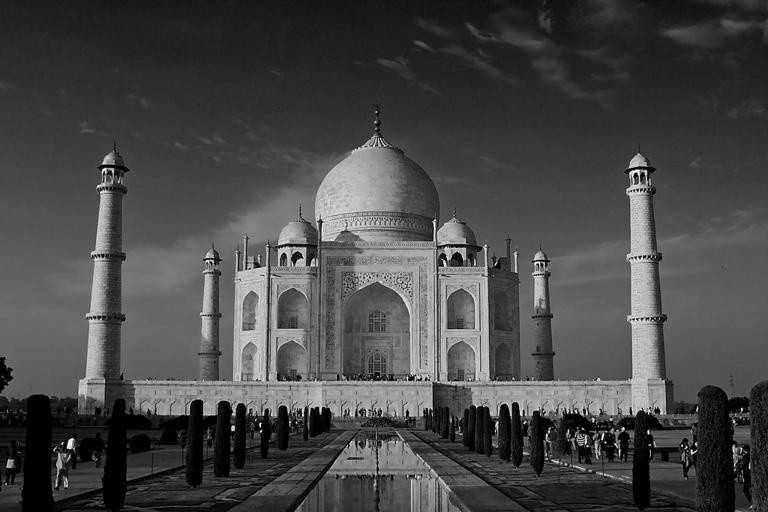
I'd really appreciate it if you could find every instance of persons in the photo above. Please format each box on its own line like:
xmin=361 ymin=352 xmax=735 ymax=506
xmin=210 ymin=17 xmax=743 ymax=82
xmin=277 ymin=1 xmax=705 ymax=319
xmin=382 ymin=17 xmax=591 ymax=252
xmin=211 ymin=425 xmax=217 ymax=442
xmin=447 ymin=406 xmax=632 ymax=463
xmin=392 ymin=408 xmax=397 ymax=417
xmin=678 ymin=418 xmax=754 ymax=512
xmin=342 ymin=406 xmax=384 ymax=418
xmin=230 ymin=423 xmax=235 ymax=440
xmin=62 ymin=404 xmax=158 ymax=415
xmin=640 ymin=406 xmax=661 ymax=415
xmin=65 ymin=433 xmax=79 ymax=469
xmin=3 ymin=438 xmax=21 ymax=484
xmin=466 ymin=374 xmax=543 ymax=382
xmin=646 ymin=429 xmax=657 ymax=462
xmin=591 ymin=375 xmax=601 ymax=382
xmin=53 ymin=440 xmax=73 ymax=491
xmin=247 ymin=416 xmax=304 ymax=440
xmin=405 ymin=408 xmax=410 ymax=417
xmin=282 ymin=371 xmax=432 ymax=381
xmin=91 ymin=430 xmax=103 ymax=467
xmin=205 ymin=426 xmax=213 ymax=448
